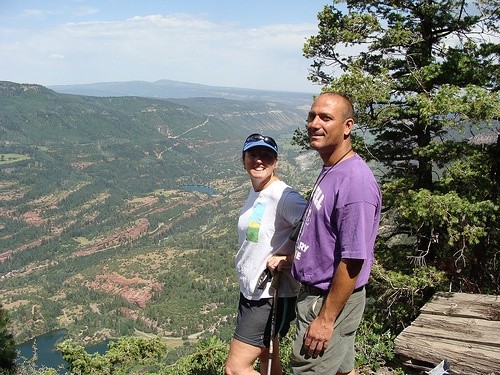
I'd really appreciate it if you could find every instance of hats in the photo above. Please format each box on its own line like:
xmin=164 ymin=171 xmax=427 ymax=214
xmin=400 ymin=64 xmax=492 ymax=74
xmin=242 ymin=137 xmax=279 ymax=154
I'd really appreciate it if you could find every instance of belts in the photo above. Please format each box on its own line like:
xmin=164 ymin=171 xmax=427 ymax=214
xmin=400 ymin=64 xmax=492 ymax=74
xmin=304 ymin=285 xmax=364 ymax=296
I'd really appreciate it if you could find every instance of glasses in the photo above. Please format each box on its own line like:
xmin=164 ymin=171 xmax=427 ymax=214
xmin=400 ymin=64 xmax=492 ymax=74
xmin=246 ymin=133 xmax=278 ymax=149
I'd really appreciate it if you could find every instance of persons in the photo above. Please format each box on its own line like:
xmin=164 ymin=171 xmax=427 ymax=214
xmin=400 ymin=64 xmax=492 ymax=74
xmin=226 ymin=136 xmax=308 ymax=375
xmin=286 ymin=92 xmax=382 ymax=375
